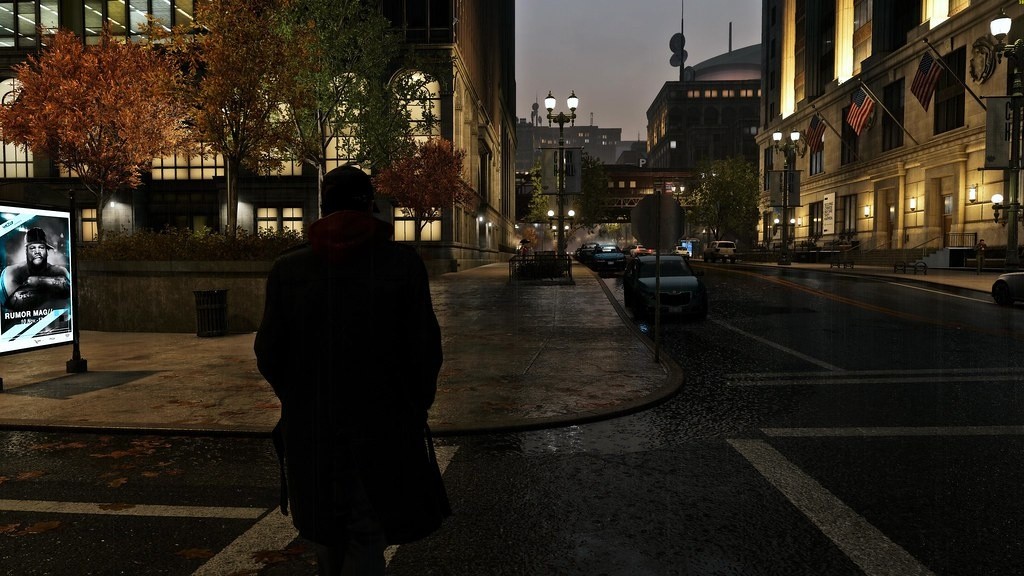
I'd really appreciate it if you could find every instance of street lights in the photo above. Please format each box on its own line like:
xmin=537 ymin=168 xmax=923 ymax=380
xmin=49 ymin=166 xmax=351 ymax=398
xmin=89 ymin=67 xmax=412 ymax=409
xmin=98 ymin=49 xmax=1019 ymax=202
xmin=773 ymin=127 xmax=800 ymax=265
xmin=543 ymin=87 xmax=578 ymax=283
xmin=989 ymin=7 xmax=1024 ymax=274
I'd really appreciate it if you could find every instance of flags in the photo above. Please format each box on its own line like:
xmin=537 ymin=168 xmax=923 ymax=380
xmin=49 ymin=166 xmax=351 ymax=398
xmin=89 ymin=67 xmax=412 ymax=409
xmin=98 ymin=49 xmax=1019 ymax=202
xmin=805 ymin=114 xmax=827 ymax=156
xmin=910 ymin=50 xmax=945 ymax=112
xmin=845 ymin=87 xmax=875 ymax=137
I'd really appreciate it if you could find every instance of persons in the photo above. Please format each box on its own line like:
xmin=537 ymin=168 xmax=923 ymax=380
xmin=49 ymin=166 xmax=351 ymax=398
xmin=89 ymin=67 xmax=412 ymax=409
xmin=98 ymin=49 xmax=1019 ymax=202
xmin=253 ymin=166 xmax=443 ymax=576
xmin=974 ymin=239 xmax=988 ymax=275
xmin=520 ymin=243 xmax=529 ymax=254
xmin=0 ymin=227 xmax=70 ymax=330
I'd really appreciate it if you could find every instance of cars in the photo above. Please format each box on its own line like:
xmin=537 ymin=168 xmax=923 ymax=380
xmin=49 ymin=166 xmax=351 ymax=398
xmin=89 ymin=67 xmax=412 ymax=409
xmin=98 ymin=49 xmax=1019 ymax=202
xmin=675 ymin=247 xmax=689 ymax=260
xmin=704 ymin=241 xmax=737 ymax=264
xmin=630 ymin=244 xmax=652 ymax=255
xmin=622 ymin=255 xmax=704 ymax=323
xmin=576 ymin=241 xmax=626 ymax=272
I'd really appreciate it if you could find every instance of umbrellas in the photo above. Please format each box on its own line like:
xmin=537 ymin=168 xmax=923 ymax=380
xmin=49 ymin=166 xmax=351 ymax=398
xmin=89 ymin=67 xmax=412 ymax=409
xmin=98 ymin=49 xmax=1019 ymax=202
xmin=520 ymin=239 xmax=530 ymax=243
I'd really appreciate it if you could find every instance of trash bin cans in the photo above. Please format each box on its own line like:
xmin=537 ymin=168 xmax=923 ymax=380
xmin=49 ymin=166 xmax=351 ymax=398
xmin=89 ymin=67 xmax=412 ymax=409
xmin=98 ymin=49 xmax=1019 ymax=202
xmin=190 ymin=286 xmax=231 ymax=338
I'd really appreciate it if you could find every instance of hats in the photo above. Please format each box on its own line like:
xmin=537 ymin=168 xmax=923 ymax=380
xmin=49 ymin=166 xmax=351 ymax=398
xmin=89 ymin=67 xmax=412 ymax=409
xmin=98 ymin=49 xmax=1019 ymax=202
xmin=24 ymin=227 xmax=55 ymax=250
xmin=520 ymin=239 xmax=530 ymax=244
